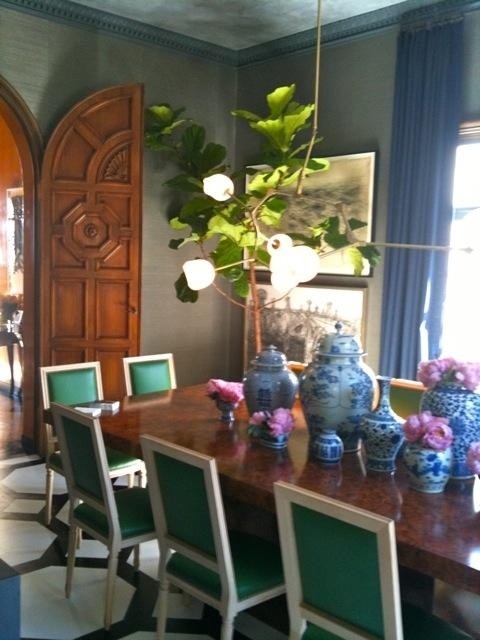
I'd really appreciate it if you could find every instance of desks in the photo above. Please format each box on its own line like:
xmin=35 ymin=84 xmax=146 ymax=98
xmin=1 ymin=332 xmax=20 ymax=399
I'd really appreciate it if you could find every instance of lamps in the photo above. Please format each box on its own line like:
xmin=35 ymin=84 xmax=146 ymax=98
xmin=183 ymin=170 xmax=322 ymax=312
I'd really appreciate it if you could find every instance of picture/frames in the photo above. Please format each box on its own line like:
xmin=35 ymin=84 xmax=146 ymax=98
xmin=241 ymin=275 xmax=370 ymax=372
xmin=238 ymin=151 xmax=379 ymax=278
